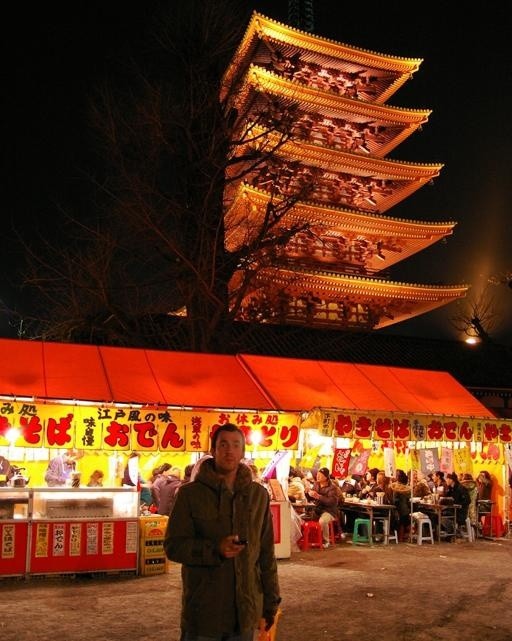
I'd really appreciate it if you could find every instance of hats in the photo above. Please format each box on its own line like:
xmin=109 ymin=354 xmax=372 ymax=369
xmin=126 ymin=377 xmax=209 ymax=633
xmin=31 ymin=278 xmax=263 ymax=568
xmin=318 ymin=468 xmax=328 ymax=478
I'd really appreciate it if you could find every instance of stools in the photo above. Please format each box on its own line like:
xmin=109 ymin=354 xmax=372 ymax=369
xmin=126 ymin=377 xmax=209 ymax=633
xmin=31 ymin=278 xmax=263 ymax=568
xmin=296 ymin=512 xmax=506 ymax=555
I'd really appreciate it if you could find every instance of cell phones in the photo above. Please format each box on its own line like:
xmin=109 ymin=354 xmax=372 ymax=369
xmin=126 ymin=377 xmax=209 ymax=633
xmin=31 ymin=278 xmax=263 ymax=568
xmin=234 ymin=539 xmax=249 ymax=545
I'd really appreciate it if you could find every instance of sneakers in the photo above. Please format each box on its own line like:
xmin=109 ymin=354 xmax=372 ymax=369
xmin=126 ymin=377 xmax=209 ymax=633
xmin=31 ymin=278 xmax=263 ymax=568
xmin=322 ymin=543 xmax=330 ymax=549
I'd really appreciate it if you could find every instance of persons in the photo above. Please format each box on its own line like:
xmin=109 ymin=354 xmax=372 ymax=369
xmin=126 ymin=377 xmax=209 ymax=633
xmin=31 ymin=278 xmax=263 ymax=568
xmin=45 ymin=448 xmax=85 ymax=489
xmin=0 ymin=454 xmax=20 ymax=523
xmin=162 ymin=424 xmax=284 ymax=641
xmin=250 ymin=465 xmax=493 ymax=549
xmin=124 ymin=452 xmax=215 ymax=517
xmin=85 ymin=470 xmax=105 ymax=488
